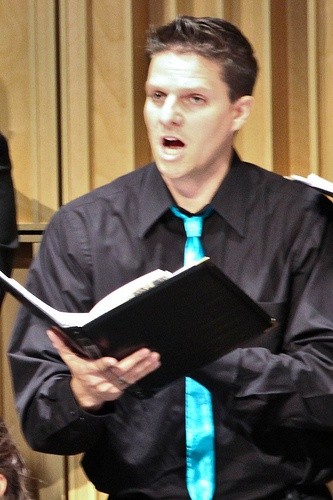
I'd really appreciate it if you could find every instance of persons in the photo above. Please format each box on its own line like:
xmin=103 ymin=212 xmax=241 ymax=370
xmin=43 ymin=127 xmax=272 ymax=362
xmin=0 ymin=129 xmax=19 ymax=312
xmin=7 ymin=16 xmax=333 ymax=500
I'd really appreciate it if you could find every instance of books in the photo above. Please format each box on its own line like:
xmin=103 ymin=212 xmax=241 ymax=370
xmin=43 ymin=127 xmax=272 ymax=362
xmin=0 ymin=254 xmax=276 ymax=401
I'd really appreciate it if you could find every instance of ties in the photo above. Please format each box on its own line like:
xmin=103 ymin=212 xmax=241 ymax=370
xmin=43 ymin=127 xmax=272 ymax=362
xmin=169 ymin=206 xmax=215 ymax=500
